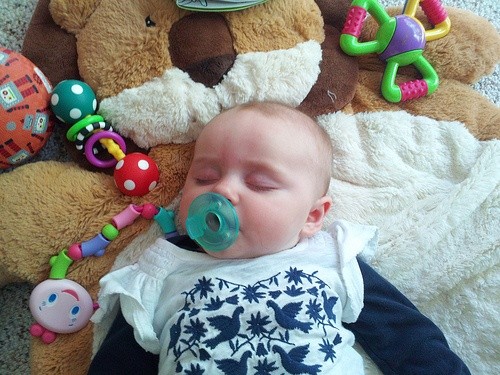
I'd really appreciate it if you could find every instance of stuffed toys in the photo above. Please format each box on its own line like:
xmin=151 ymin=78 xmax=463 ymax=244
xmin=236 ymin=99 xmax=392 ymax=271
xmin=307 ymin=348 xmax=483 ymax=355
xmin=1 ymin=0 xmax=500 ymax=375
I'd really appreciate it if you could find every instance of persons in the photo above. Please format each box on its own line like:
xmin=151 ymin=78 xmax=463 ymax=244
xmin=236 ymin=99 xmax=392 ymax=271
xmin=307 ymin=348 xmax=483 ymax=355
xmin=86 ymin=101 xmax=471 ymax=375
